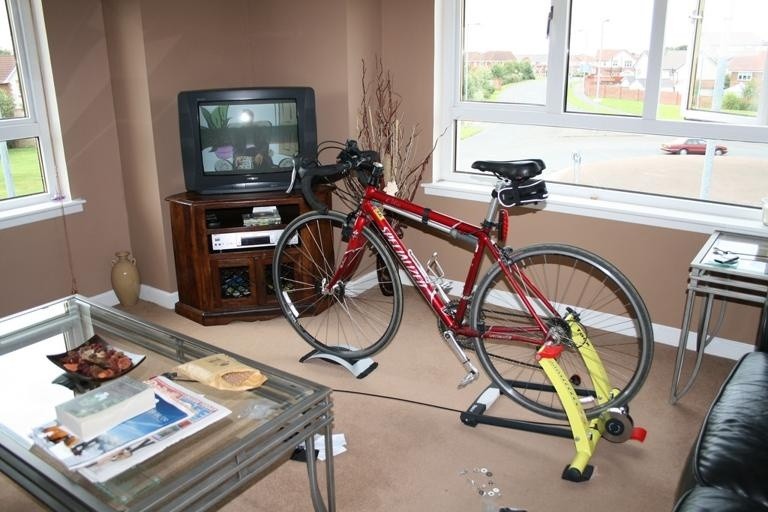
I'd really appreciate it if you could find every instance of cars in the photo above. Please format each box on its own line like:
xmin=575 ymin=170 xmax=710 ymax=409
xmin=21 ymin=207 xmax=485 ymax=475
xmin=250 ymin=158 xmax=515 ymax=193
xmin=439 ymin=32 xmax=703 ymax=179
xmin=659 ymin=138 xmax=728 ymax=156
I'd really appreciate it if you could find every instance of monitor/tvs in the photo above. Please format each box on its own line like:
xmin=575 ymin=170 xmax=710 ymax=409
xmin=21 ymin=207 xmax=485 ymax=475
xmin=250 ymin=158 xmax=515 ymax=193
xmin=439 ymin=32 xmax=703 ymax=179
xmin=178 ymin=85 xmax=319 ymax=194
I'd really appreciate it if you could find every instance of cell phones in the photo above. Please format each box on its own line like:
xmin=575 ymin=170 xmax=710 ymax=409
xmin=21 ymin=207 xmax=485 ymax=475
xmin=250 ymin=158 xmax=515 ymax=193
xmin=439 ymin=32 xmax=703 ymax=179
xmin=715 ymin=253 xmax=739 ymax=263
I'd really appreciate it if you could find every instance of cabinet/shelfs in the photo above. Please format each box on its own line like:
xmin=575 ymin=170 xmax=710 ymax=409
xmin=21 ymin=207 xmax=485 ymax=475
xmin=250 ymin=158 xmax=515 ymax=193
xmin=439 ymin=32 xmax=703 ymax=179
xmin=164 ymin=186 xmax=339 ymax=326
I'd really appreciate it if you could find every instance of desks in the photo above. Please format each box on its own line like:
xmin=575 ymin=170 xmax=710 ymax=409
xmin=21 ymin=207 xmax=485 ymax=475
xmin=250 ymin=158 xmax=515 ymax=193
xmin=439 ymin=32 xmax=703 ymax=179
xmin=668 ymin=229 xmax=768 ymax=406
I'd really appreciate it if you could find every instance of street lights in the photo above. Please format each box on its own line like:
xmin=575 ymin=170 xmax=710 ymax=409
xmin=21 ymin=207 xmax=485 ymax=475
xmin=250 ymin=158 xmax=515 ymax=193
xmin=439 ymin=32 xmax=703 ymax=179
xmin=593 ymin=18 xmax=611 ymax=107
xmin=464 ymin=23 xmax=481 ymax=101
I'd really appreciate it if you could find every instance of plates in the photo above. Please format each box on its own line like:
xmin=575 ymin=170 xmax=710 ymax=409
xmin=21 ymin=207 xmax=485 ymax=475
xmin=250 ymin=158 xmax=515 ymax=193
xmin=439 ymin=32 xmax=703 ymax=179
xmin=48 ymin=336 xmax=144 ymax=379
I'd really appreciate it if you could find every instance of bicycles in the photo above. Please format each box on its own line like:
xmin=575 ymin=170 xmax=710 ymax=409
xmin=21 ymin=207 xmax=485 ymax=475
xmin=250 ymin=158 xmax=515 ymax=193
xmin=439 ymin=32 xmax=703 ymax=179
xmin=271 ymin=139 xmax=654 ymax=421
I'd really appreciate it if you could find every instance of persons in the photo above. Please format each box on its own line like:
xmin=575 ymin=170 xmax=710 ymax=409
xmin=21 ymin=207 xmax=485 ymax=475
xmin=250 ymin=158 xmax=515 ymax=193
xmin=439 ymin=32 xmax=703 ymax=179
xmin=232 ymin=107 xmax=274 ymax=170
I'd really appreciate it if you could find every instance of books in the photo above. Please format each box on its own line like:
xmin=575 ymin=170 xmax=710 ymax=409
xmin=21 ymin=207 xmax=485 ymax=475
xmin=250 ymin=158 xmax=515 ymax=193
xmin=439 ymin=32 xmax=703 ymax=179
xmin=30 ymin=385 xmax=193 ymax=472
xmin=54 ymin=373 xmax=157 ymax=442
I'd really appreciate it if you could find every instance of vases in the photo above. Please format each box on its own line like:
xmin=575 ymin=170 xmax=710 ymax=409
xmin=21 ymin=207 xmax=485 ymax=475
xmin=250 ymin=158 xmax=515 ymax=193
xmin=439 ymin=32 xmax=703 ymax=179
xmin=376 ymin=215 xmax=407 ymax=296
xmin=109 ymin=251 xmax=142 ymax=307
